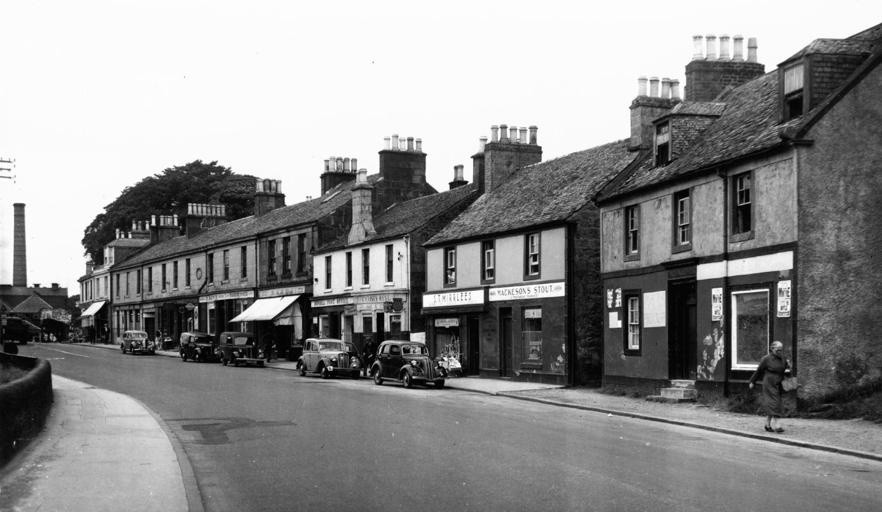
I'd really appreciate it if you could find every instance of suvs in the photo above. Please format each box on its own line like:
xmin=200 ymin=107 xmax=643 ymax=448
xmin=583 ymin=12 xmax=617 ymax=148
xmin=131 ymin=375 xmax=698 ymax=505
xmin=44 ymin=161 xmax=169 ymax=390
xmin=178 ymin=331 xmax=220 ymax=363
xmin=294 ymin=336 xmax=362 ymax=380
xmin=119 ymin=329 xmax=157 ymax=356
xmin=370 ymin=337 xmax=446 ymax=389
xmin=218 ymin=329 xmax=266 ymax=367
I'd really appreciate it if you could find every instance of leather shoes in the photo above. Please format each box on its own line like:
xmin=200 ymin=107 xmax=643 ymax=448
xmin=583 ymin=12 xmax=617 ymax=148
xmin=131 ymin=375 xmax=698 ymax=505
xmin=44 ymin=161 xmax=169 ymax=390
xmin=764 ymin=425 xmax=783 ymax=433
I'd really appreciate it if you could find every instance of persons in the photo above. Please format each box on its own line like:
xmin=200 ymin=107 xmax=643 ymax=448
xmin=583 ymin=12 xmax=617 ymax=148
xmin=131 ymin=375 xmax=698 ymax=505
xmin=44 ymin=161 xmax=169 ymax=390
xmin=748 ymin=340 xmax=793 ymax=434
xmin=155 ymin=327 xmax=163 ymax=350
xmin=90 ymin=324 xmax=96 ymax=344
xmin=102 ymin=323 xmax=110 ymax=344
xmin=362 ymin=337 xmax=376 ymax=377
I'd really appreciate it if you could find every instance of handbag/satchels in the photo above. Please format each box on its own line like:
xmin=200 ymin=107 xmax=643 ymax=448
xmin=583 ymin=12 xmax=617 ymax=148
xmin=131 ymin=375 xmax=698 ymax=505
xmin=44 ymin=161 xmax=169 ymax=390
xmin=782 ymin=376 xmax=798 ymax=391
